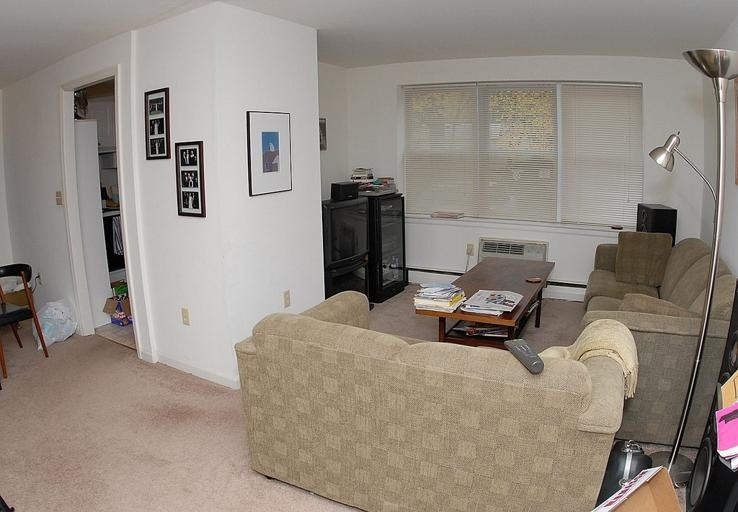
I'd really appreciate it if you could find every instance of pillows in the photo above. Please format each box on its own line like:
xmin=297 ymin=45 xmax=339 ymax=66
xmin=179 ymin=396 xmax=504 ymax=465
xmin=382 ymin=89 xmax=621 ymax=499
xmin=616 ymin=231 xmax=672 ymax=287
xmin=618 ymin=293 xmax=702 ymax=319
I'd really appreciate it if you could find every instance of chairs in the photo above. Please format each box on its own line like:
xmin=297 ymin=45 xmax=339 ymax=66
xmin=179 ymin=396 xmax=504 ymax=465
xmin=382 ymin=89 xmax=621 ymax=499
xmin=0 ymin=263 xmax=49 ymax=378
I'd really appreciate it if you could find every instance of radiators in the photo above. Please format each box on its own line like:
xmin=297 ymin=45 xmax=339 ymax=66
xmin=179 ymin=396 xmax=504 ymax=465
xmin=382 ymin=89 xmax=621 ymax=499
xmin=477 ymin=237 xmax=549 ymax=264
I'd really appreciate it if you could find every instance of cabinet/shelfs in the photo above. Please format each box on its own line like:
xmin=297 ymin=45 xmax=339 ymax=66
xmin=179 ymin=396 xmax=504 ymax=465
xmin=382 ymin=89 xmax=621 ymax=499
xmin=325 ymin=263 xmax=374 ymax=311
xmin=359 ymin=191 xmax=409 ymax=303
xmin=73 ymin=97 xmax=126 ymax=329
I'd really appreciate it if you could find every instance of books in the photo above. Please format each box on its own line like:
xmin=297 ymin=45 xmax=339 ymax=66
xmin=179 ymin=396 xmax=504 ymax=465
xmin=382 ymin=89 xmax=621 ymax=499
xmin=348 ymin=167 xmax=399 ymax=197
xmin=431 ymin=211 xmax=464 ymax=219
xmin=412 ymin=281 xmax=526 ymax=339
xmin=715 ymin=369 xmax=738 ymax=472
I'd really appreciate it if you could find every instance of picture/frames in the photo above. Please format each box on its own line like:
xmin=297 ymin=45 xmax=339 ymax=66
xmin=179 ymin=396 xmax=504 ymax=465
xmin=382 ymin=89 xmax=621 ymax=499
xmin=246 ymin=111 xmax=292 ymax=197
xmin=175 ymin=141 xmax=206 ymax=217
xmin=144 ymin=87 xmax=171 ymax=160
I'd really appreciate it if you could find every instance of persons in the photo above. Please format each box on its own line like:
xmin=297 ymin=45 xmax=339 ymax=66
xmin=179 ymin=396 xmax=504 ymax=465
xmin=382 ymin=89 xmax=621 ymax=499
xmin=149 ymin=98 xmax=165 ymax=156
xmin=182 ymin=149 xmax=198 ymax=209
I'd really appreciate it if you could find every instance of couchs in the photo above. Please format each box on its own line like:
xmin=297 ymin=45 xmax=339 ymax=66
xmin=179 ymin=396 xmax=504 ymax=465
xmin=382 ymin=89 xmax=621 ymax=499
xmin=235 ymin=291 xmax=638 ymax=512
xmin=581 ymin=238 xmax=736 ymax=447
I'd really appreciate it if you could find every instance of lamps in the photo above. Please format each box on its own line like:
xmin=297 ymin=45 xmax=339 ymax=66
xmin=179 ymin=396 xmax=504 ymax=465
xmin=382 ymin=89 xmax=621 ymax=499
xmin=648 ymin=48 xmax=738 ymax=490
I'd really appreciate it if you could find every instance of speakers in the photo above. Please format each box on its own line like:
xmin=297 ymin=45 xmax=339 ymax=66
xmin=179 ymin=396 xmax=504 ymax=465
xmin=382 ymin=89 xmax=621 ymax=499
xmin=331 ymin=182 xmax=358 ymax=201
xmin=685 ymin=279 xmax=738 ymax=512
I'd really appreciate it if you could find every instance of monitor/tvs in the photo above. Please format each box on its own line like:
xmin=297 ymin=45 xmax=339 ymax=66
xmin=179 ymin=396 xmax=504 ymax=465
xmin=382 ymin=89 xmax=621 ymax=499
xmin=322 ymin=197 xmax=370 ymax=271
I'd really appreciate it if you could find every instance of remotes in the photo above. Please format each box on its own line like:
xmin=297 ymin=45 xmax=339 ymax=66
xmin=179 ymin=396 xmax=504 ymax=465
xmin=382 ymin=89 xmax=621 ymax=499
xmin=503 ymin=339 xmax=545 ymax=375
